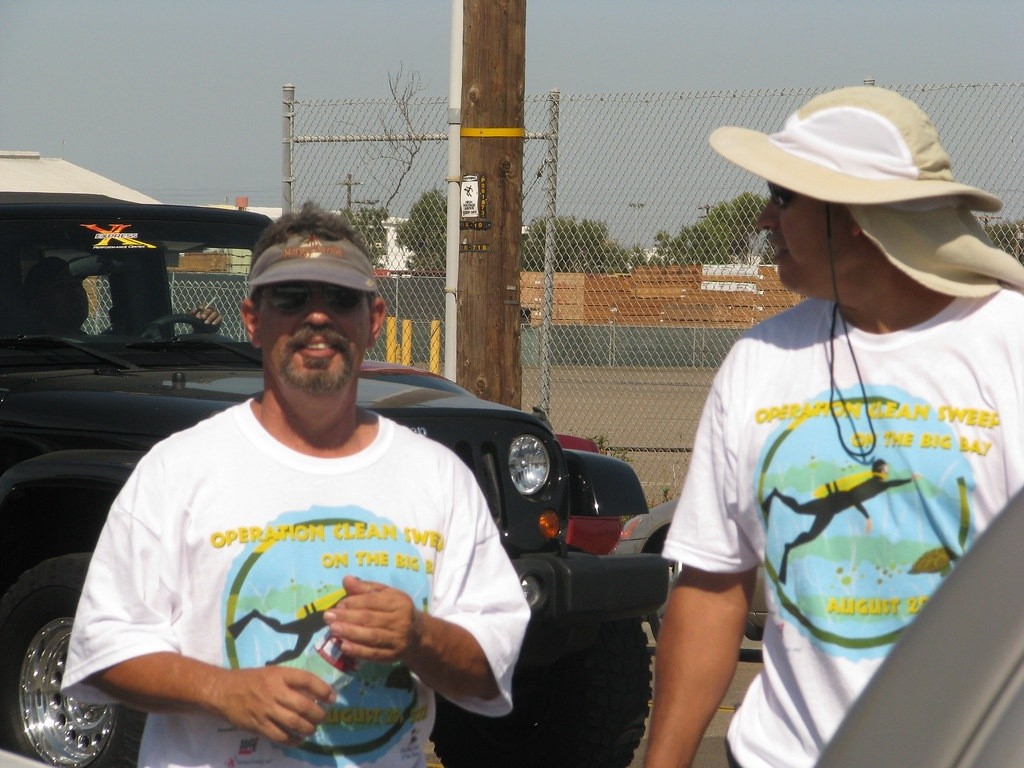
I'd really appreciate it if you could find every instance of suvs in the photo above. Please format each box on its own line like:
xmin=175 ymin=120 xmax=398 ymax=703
xmin=0 ymin=186 xmax=671 ymax=768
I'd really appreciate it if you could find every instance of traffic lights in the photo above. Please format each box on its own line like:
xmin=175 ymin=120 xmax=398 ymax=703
xmin=428 ymin=321 xmax=446 ymax=375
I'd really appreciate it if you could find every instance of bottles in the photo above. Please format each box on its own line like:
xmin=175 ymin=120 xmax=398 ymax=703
xmin=293 ymin=626 xmax=384 ymax=741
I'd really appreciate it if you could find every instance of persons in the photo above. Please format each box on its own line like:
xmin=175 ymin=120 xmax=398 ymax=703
xmin=61 ymin=199 xmax=532 ymax=768
xmin=643 ymin=86 xmax=1024 ymax=768
xmin=17 ymin=256 xmax=223 ymax=337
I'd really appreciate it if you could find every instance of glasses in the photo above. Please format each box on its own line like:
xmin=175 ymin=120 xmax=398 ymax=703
xmin=768 ymin=182 xmax=794 ymax=210
xmin=257 ymin=280 xmax=376 ymax=312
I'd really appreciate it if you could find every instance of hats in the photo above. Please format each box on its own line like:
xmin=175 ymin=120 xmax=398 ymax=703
xmin=24 ymin=256 xmax=90 ymax=297
xmin=710 ymin=86 xmax=1024 ymax=298
xmin=249 ymin=235 xmax=377 ymax=306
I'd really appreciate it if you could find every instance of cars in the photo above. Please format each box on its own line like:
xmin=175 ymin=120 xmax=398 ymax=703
xmin=609 ymin=494 xmax=769 ymax=646
xmin=353 ymin=357 xmax=648 ymax=556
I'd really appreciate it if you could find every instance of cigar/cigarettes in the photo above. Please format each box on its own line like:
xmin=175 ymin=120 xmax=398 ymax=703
xmin=198 ymin=297 xmax=217 ymax=314
